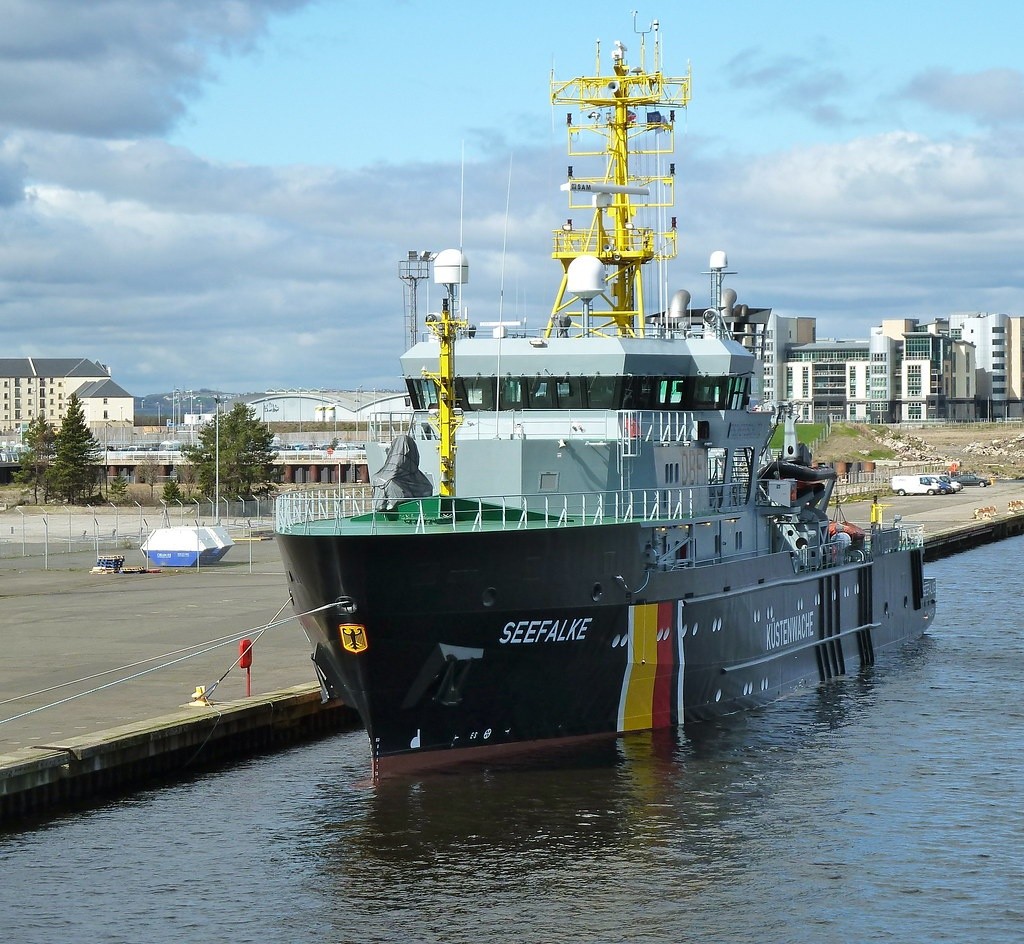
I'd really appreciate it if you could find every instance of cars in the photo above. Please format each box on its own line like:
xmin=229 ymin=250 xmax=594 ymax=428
xmin=952 ymin=474 xmax=991 ymax=487
xmin=91 ymin=444 xmax=367 ymax=459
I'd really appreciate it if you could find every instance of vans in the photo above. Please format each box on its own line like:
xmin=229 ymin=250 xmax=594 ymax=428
xmin=892 ymin=475 xmax=963 ymax=495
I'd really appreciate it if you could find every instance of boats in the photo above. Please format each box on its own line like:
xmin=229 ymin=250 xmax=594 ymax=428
xmin=274 ymin=7 xmax=941 ymax=783
